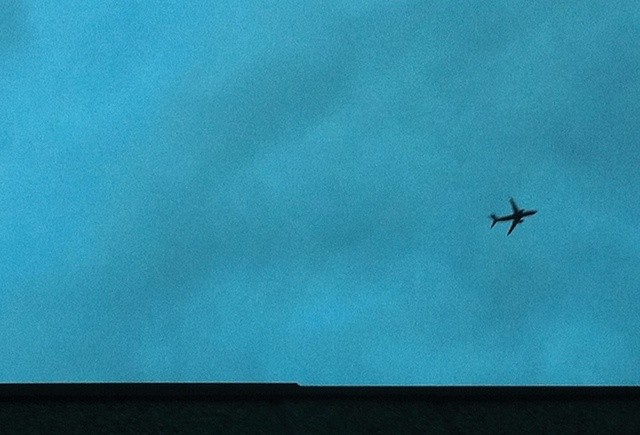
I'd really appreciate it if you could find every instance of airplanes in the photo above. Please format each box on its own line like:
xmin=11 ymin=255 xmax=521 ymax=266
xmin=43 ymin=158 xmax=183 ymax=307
xmin=490 ymin=199 xmax=537 ymax=235
